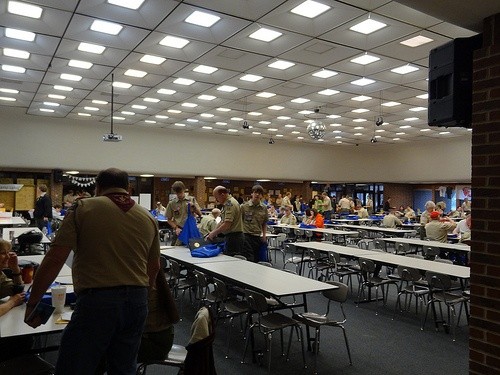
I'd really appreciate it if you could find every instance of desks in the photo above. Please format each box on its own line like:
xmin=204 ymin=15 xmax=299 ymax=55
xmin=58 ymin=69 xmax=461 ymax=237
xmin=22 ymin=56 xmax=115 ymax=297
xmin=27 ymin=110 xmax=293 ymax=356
xmin=352 ymin=249 xmax=470 ymax=279
xmin=2 ymin=227 xmax=51 ymax=253
xmin=0 ymin=216 xmax=26 ymax=227
xmin=270 ymin=224 xmax=360 ymax=246
xmin=0 ymin=304 xmax=75 ymax=339
xmin=331 ymin=218 xmax=378 ymax=223
xmin=377 ymin=237 xmax=471 ymax=266
xmin=192 ymin=259 xmax=340 ymax=364
xmin=400 ymin=222 xmax=423 ymax=227
xmin=284 ymin=241 xmax=383 ymax=278
xmin=323 ymin=225 xmax=416 ymax=242
xmin=159 ymin=246 xmax=241 ymax=264
xmin=0 ymin=212 xmax=12 ymax=217
xmin=17 ymin=253 xmax=75 ymax=295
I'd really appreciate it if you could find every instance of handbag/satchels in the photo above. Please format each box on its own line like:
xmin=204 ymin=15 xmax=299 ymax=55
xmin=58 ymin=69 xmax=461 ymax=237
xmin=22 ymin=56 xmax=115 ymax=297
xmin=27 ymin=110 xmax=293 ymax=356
xmin=11 ymin=230 xmax=43 ymax=252
xmin=260 ymin=242 xmax=268 ymax=262
xmin=24 ymin=282 xmax=76 ymax=306
xmin=191 ymin=244 xmax=222 ymax=258
xmin=44 ymin=221 xmax=52 ymax=235
xmin=178 ymin=203 xmax=203 ymax=245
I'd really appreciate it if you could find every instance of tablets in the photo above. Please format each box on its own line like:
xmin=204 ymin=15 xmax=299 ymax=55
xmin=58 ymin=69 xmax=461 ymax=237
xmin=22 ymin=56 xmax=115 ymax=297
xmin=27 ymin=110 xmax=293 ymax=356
xmin=24 ymin=301 xmax=56 ymax=325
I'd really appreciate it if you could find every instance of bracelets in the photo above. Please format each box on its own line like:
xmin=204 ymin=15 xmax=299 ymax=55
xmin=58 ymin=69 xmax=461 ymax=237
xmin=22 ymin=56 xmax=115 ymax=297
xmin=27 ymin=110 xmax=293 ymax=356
xmin=26 ymin=301 xmax=37 ymax=308
xmin=174 ymin=225 xmax=178 ymax=230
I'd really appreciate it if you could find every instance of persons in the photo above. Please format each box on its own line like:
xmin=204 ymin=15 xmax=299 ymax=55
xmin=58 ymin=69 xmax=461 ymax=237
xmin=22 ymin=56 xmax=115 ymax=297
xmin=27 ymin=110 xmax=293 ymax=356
xmin=164 ymin=181 xmax=202 ymax=246
xmin=420 ymin=198 xmax=471 ymax=259
xmin=208 ymin=186 xmax=245 ymax=257
xmin=383 ymin=197 xmax=415 ymax=238
xmin=260 ymin=192 xmax=278 ymax=217
xmin=33 ymin=184 xmax=52 ymax=231
xmin=236 ymin=185 xmax=268 ymax=263
xmin=155 ymin=202 xmax=166 ymax=212
xmin=198 ymin=208 xmax=221 ymax=243
xmin=334 ymin=194 xmax=373 ymax=225
xmin=276 ymin=192 xmax=333 ymax=241
xmin=0 ymin=238 xmax=25 ymax=316
xmin=43 ymin=205 xmax=62 ymax=235
xmin=135 ymin=264 xmax=181 ymax=363
xmin=64 ymin=189 xmax=91 ymax=206
xmin=24 ymin=168 xmax=161 ymax=375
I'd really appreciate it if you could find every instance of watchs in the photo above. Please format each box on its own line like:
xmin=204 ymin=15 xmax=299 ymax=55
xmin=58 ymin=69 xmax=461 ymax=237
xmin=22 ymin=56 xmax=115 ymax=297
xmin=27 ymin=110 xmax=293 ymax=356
xmin=11 ymin=267 xmax=24 ymax=276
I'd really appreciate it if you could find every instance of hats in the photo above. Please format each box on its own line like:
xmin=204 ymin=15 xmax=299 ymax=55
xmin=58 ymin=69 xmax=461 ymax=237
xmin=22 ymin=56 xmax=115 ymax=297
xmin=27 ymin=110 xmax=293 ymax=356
xmin=430 ymin=212 xmax=442 ymax=218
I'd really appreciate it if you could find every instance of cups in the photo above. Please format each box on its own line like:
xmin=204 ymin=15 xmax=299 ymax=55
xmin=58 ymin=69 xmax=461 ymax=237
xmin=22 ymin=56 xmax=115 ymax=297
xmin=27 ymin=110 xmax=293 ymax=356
xmin=51 ymin=286 xmax=66 ymax=313
xmin=24 ymin=266 xmax=34 ymax=284
xmin=9 ymin=231 xmax=14 ymax=240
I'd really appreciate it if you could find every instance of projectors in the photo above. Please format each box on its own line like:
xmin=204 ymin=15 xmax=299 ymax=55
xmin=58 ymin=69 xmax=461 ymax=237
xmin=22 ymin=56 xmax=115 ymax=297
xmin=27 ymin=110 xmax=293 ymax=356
xmin=102 ymin=134 xmax=123 ymax=142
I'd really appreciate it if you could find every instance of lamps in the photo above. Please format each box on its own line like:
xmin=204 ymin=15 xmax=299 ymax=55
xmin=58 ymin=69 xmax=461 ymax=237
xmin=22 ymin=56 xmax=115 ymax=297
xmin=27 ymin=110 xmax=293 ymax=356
xmin=268 ymin=138 xmax=275 ymax=145
xmin=102 ymin=73 xmax=122 ymax=143
xmin=306 ymin=107 xmax=327 ymax=141
xmin=375 ymin=91 xmax=384 ymax=126
xmin=371 ymin=138 xmax=377 ymax=144
xmin=243 ymin=97 xmax=249 ymax=130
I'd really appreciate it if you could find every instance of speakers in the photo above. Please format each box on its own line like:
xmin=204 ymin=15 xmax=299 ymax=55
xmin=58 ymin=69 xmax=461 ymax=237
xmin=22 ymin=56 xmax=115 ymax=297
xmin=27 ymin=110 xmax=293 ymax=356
xmin=427 ymin=37 xmax=472 ymax=128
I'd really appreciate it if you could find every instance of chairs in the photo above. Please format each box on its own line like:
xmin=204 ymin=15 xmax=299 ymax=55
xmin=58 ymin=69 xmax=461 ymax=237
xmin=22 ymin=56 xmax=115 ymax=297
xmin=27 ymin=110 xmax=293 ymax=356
xmin=0 ymin=210 xmax=471 ymax=375
